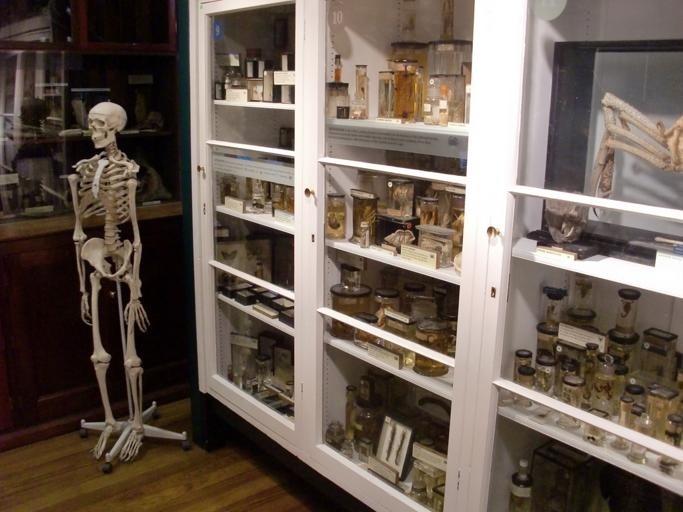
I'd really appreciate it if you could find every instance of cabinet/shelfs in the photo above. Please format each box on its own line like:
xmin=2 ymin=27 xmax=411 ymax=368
xmin=189 ymin=0 xmax=683 ymax=512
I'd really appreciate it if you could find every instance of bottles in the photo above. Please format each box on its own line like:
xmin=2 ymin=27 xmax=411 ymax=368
xmin=215 ymin=39 xmax=296 ymax=106
xmin=331 ymin=260 xmax=458 ymax=375
xmin=512 ymin=271 xmax=683 ymax=471
xmin=332 ymin=380 xmax=379 ymax=465
xmin=331 ymin=28 xmax=470 ymax=128
xmin=505 ymin=452 xmax=536 ymax=509
xmin=214 ymin=151 xmax=295 ymax=221
xmin=324 ymin=148 xmax=466 ymax=266
